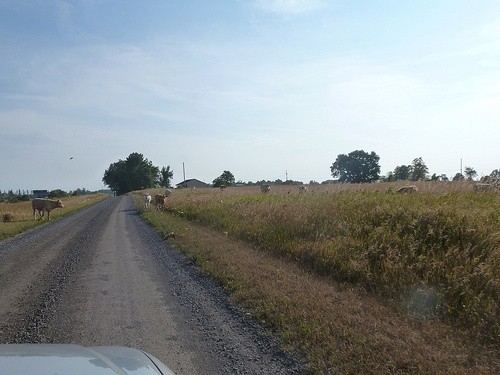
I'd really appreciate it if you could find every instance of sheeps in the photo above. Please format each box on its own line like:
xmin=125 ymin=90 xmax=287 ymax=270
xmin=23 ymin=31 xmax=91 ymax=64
xmin=32 ymin=198 xmax=64 ymax=221
xmin=154 ymin=193 xmax=167 ymax=211
xmin=144 ymin=192 xmax=151 ymax=209
